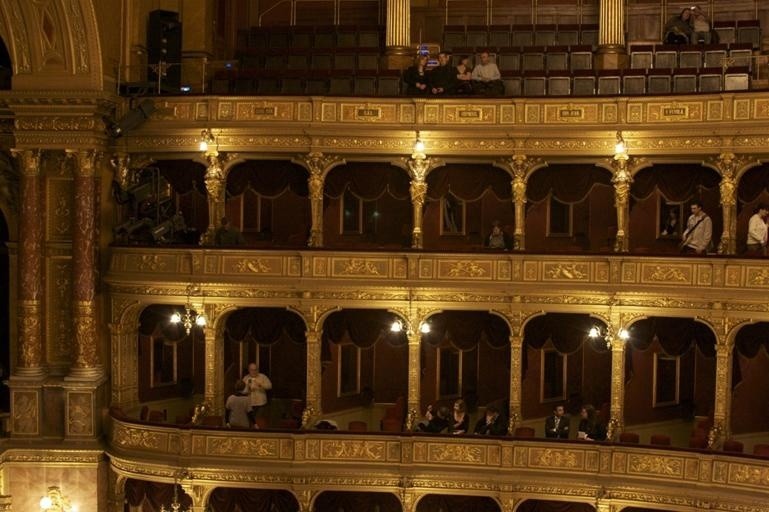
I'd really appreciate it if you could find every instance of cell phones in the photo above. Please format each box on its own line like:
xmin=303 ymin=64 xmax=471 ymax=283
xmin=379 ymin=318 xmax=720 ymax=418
xmin=690 ymin=6 xmax=696 ymax=10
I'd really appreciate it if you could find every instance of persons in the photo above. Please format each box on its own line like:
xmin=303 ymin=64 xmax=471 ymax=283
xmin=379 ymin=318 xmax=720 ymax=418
xmin=415 ymin=397 xmax=606 ymax=442
xmin=745 ymin=203 xmax=769 ymax=255
xmin=682 ymin=199 xmax=713 ymax=256
xmin=216 ymin=217 xmax=246 ymax=245
xmin=243 ymin=362 xmax=273 ymax=430
xmin=661 ymin=208 xmax=680 ymax=236
xmin=484 ymin=219 xmax=512 ymax=249
xmin=405 ymin=49 xmax=506 ymax=98
xmin=664 ymin=5 xmax=713 ymax=44
xmin=226 ymin=379 xmax=253 ymax=428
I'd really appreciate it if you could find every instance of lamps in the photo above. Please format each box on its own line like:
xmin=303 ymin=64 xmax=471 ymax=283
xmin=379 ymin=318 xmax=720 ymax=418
xmin=411 ymin=130 xmax=427 ymax=160
xmin=390 ymin=283 xmax=432 ymax=340
xmin=39 ymin=485 xmax=78 ymax=512
xmin=197 ymin=129 xmax=215 ymax=151
xmin=614 ymin=128 xmax=629 ymax=161
xmin=590 ymin=294 xmax=630 ymax=348
xmin=171 ymin=283 xmax=208 ymax=335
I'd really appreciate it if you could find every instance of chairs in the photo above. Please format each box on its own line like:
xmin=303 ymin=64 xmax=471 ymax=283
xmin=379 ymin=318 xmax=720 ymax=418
xmin=226 ymin=21 xmax=768 ymax=96
xmin=234 ymin=232 xmax=769 ymax=261
xmin=107 ymin=395 xmax=769 ymax=459
